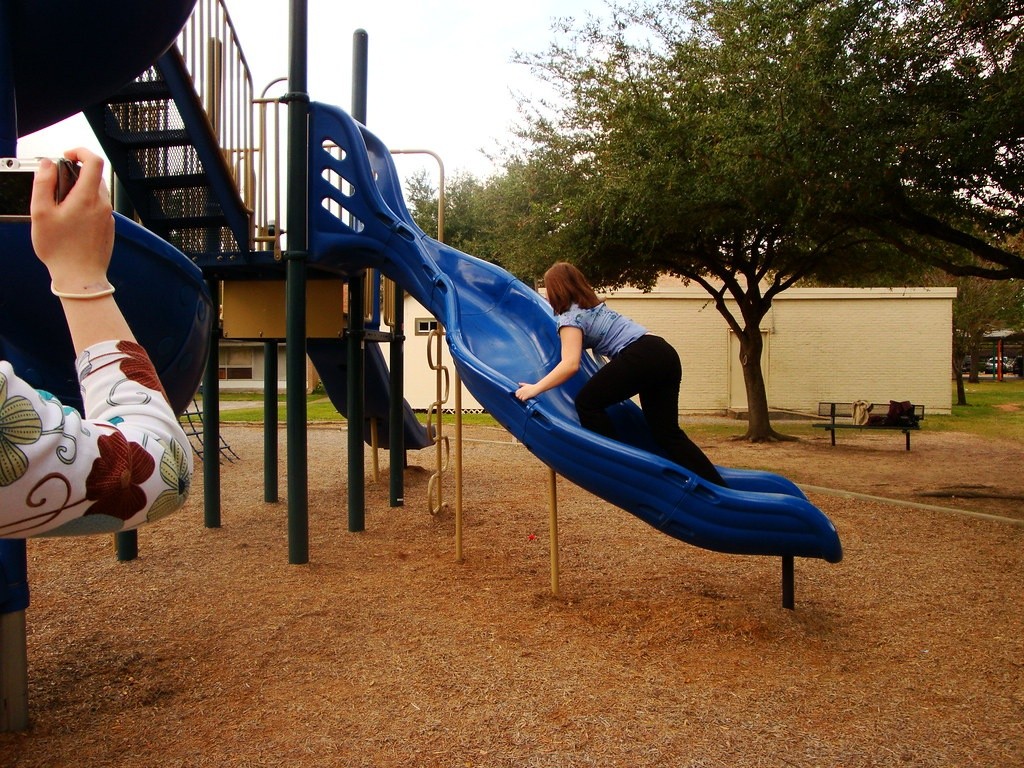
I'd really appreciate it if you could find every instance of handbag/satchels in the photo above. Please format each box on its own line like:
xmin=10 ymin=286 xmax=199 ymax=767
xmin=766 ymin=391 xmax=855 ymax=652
xmin=851 ymin=400 xmax=873 ymax=425
xmin=883 ymin=400 xmax=920 ymax=427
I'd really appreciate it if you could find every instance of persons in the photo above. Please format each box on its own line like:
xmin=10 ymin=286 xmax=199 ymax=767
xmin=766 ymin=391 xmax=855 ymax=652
xmin=0 ymin=148 xmax=193 ymax=539
xmin=515 ymin=260 xmax=731 ymax=489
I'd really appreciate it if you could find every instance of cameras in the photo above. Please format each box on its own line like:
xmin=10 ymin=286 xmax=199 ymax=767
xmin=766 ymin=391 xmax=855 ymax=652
xmin=1 ymin=156 xmax=81 ymax=223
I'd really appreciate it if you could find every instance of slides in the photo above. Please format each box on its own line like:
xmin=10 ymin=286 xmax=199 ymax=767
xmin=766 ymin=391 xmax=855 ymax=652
xmin=303 ymin=97 xmax=851 ymax=566
xmin=303 ymin=339 xmax=435 ymax=450
xmin=0 ymin=1 xmax=215 ymax=616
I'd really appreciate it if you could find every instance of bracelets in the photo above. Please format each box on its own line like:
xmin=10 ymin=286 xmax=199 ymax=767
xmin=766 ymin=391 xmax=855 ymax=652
xmin=51 ymin=279 xmax=115 ymax=298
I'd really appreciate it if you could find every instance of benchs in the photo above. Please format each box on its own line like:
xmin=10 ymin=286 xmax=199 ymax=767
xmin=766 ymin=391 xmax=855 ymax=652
xmin=812 ymin=402 xmax=925 ymax=451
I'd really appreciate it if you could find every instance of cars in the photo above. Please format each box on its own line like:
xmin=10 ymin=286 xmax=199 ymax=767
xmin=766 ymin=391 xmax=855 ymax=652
xmin=984 ymin=356 xmax=1024 ymax=375
xmin=961 ymin=354 xmax=972 ymax=372
